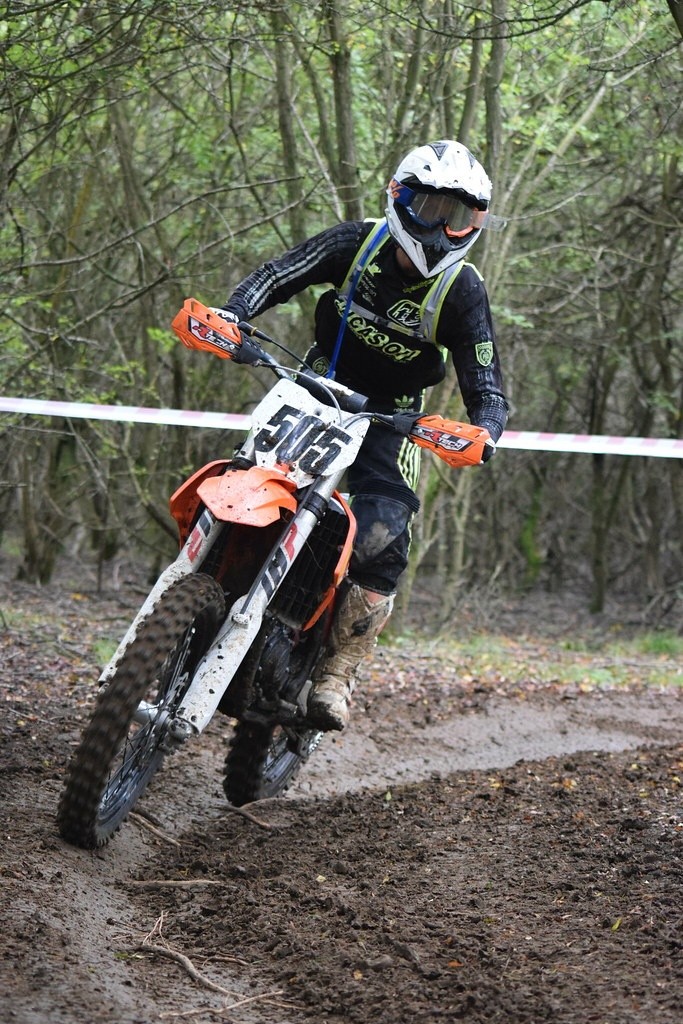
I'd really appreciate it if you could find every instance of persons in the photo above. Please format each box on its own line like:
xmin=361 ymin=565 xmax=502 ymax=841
xmin=219 ymin=139 xmax=509 ymax=732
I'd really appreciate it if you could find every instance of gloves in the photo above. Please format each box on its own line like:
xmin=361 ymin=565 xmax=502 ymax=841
xmin=207 ymin=304 xmax=242 ymax=326
xmin=480 ymin=438 xmax=497 ymax=465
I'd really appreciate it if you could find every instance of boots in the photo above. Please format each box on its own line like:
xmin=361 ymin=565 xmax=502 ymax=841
xmin=307 ymin=576 xmax=397 ymax=732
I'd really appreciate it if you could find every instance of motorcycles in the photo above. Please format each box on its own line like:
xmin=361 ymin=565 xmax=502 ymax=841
xmin=52 ymin=299 xmax=499 ymax=851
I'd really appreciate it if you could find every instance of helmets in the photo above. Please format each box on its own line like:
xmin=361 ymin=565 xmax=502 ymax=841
xmin=383 ymin=139 xmax=493 ymax=279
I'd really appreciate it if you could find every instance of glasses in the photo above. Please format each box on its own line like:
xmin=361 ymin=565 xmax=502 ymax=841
xmin=387 ymin=178 xmax=490 ymax=251
